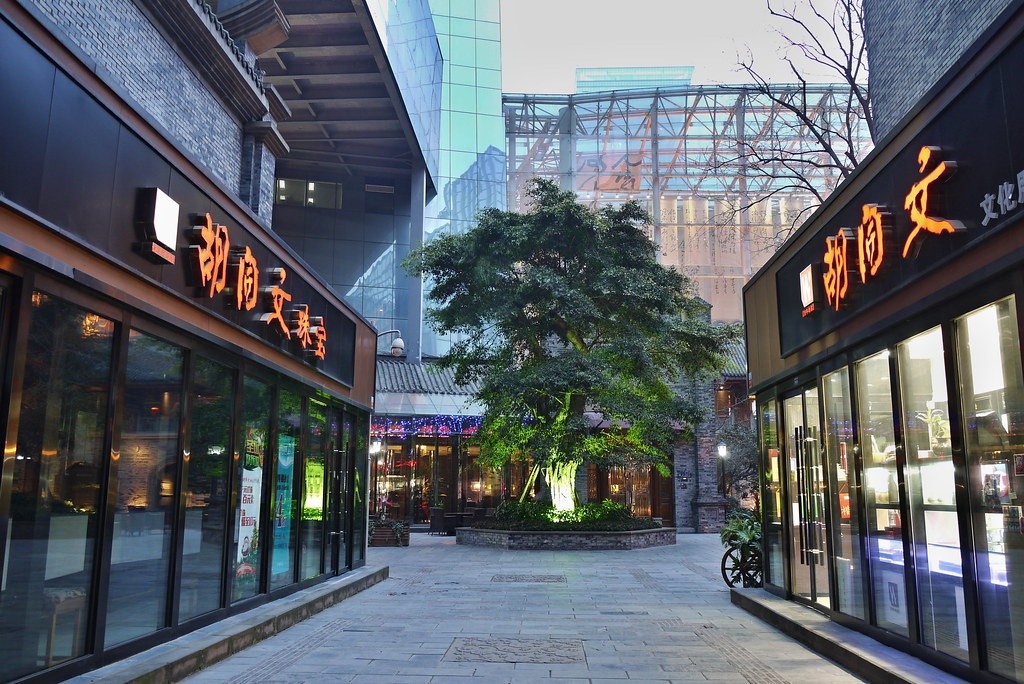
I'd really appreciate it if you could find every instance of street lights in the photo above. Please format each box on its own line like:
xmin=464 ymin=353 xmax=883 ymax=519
xmin=717 ymin=442 xmax=727 ymax=524
xmin=372 ymin=437 xmax=382 ymax=514
